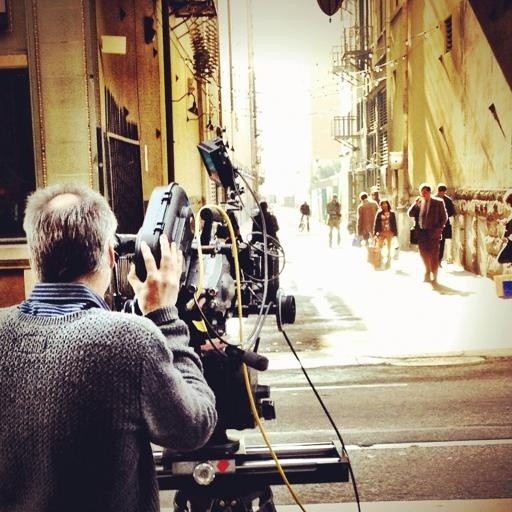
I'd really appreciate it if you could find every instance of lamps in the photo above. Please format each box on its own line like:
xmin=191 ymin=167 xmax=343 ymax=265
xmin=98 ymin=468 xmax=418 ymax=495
xmin=186 ymin=113 xmax=214 ymax=131
xmin=172 ymin=93 xmax=199 ymax=116
xmin=366 ymin=152 xmax=384 ymax=171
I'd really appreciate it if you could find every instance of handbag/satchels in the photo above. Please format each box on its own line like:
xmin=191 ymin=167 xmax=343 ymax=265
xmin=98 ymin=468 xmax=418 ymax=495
xmin=494 ymin=274 xmax=512 ymax=297
xmin=368 ymin=246 xmax=381 ymax=267
xmin=410 ymin=226 xmax=419 ymax=244
xmin=502 ymin=281 xmax=512 ymax=297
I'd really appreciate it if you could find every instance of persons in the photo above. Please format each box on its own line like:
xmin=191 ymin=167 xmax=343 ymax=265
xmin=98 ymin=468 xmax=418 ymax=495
xmin=354 ymin=192 xmax=379 ymax=251
xmin=250 ymin=202 xmax=279 ymax=299
xmin=298 ymin=201 xmax=311 ymax=232
xmin=494 ymin=191 xmax=512 ymax=287
xmin=371 ymin=190 xmax=380 ymax=209
xmin=1 ymin=177 xmax=219 ymax=512
xmin=325 ymin=193 xmax=342 ymax=249
xmin=406 ymin=184 xmax=448 ymax=287
xmin=373 ymin=200 xmax=398 ymax=271
xmin=429 ymin=185 xmax=456 ymax=270
